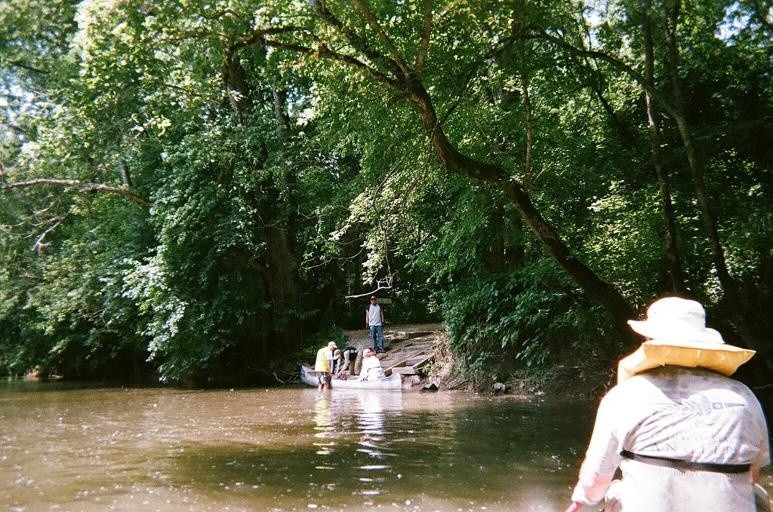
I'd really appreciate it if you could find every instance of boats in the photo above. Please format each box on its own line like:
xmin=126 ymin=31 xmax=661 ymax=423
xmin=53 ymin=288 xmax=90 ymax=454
xmin=296 ymin=364 xmax=403 ymax=391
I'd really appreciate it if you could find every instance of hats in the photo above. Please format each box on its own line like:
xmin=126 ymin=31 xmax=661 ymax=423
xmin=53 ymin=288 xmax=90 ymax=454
xmin=328 ymin=341 xmax=337 ymax=348
xmin=371 ymin=296 xmax=376 ymax=300
xmin=627 ymin=297 xmax=726 ymax=344
xmin=363 ymin=349 xmax=370 ymax=354
xmin=333 ymin=349 xmax=341 ymax=359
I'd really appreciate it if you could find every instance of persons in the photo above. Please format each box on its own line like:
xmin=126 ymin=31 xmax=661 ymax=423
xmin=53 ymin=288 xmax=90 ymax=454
xmin=563 ymin=296 xmax=770 ymax=512
xmin=314 ymin=296 xmax=385 ymax=391
